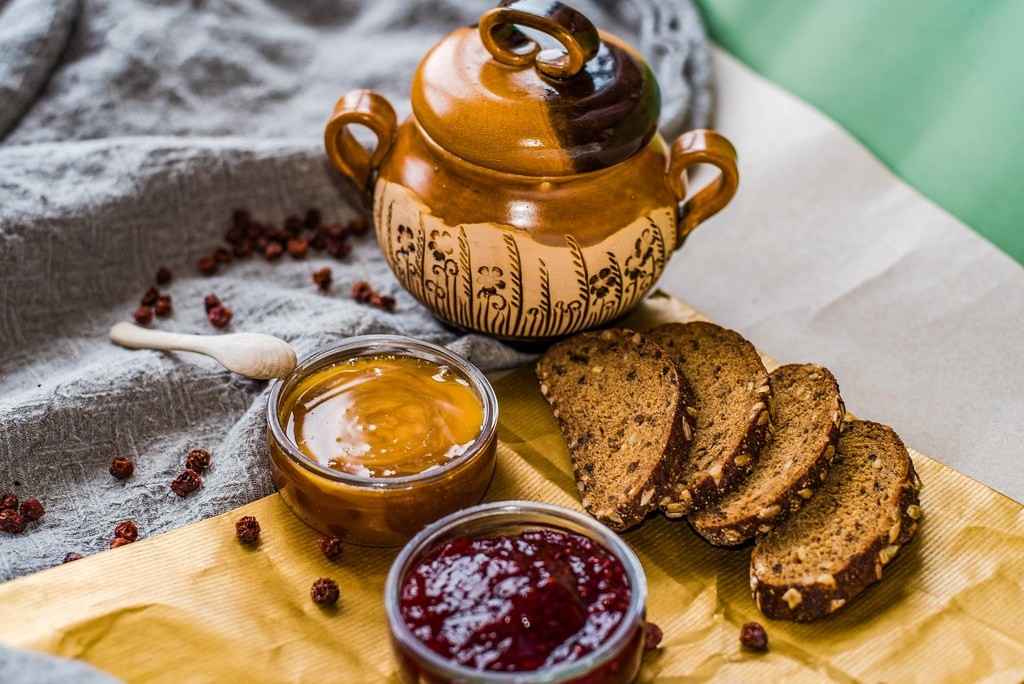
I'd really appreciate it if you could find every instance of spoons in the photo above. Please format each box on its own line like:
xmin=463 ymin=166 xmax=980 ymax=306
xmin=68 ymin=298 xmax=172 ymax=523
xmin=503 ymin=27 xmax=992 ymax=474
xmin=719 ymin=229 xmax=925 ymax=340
xmin=107 ymin=321 xmax=297 ymax=379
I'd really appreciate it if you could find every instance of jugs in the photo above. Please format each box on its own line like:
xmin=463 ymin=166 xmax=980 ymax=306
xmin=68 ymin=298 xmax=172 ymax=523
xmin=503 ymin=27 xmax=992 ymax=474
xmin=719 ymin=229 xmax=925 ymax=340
xmin=323 ymin=0 xmax=740 ymax=356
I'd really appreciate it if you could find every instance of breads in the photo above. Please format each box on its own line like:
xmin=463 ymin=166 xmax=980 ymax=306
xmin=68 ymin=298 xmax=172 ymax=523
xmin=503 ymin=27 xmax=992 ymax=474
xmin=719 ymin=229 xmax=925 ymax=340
xmin=749 ymin=420 xmax=924 ymax=622
xmin=535 ymin=327 xmax=698 ymax=532
xmin=688 ymin=363 xmax=846 ymax=546
xmin=640 ymin=321 xmax=775 ymax=520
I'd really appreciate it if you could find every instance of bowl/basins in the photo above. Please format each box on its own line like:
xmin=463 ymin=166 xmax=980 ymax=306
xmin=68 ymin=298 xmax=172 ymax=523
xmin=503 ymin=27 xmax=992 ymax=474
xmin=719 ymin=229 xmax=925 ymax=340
xmin=383 ymin=498 xmax=647 ymax=684
xmin=265 ymin=332 xmax=499 ymax=547
